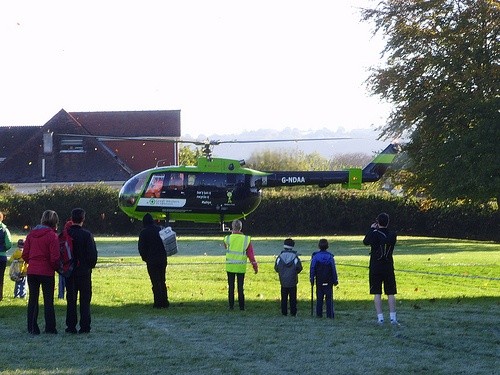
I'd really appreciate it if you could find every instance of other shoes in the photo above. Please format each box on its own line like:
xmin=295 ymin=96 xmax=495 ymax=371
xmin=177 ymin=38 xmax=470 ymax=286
xmin=78 ymin=328 xmax=89 ymax=333
xmin=42 ymin=330 xmax=56 ymax=334
xmin=391 ymin=319 xmax=398 ymax=324
xmin=378 ymin=319 xmax=383 ymax=323
xmin=65 ymin=327 xmax=76 ymax=333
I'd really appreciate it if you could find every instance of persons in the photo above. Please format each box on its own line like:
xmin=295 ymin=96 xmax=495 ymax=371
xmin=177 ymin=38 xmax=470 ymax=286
xmin=22 ymin=210 xmax=61 ymax=334
xmin=143 ymin=173 xmax=163 ymax=197
xmin=58 ymin=272 xmax=65 ymax=299
xmin=310 ymin=239 xmax=338 ymax=318
xmin=9 ymin=238 xmax=29 ymax=298
xmin=138 ymin=213 xmax=169 ymax=308
xmin=363 ymin=213 xmax=401 ymax=327
xmin=224 ymin=219 xmax=258 ymax=311
xmin=170 ymin=173 xmax=182 ymax=190
xmin=0 ymin=211 xmax=12 ymax=301
xmin=274 ymin=239 xmax=303 ymax=316
xmin=58 ymin=208 xmax=98 ymax=334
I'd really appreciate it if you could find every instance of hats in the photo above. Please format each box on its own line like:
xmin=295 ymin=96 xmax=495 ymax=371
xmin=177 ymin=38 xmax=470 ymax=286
xmin=284 ymin=239 xmax=295 ymax=249
xmin=18 ymin=239 xmax=24 ymax=248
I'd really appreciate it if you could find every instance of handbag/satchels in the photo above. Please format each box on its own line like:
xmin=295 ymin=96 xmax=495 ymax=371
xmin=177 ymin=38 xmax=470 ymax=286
xmin=158 ymin=225 xmax=179 ymax=257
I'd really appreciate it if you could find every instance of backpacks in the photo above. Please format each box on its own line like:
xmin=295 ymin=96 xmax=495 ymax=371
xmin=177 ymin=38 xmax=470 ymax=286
xmin=9 ymin=254 xmax=25 ymax=281
xmin=55 ymin=234 xmax=80 ymax=278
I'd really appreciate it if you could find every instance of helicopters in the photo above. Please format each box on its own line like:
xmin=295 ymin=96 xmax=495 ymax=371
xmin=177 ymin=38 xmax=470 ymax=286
xmin=52 ymin=132 xmax=400 ymax=233
